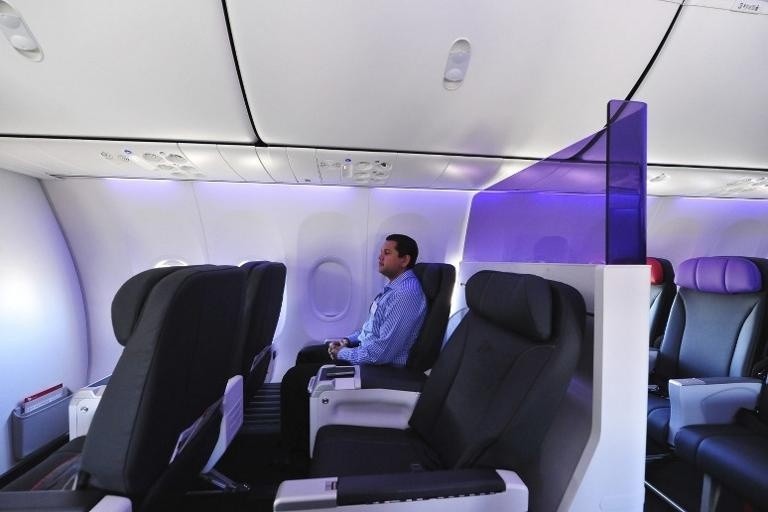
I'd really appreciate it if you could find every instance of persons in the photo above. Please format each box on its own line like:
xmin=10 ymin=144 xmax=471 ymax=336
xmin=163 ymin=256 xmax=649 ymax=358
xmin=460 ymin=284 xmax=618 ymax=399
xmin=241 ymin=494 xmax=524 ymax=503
xmin=265 ymin=234 xmax=428 ymax=458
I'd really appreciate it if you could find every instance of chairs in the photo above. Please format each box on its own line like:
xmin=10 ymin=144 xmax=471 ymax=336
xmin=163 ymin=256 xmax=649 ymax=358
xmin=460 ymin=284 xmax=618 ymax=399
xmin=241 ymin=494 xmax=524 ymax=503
xmin=646 ymin=254 xmax=768 ymax=512
xmin=309 ymin=262 xmax=455 ymax=459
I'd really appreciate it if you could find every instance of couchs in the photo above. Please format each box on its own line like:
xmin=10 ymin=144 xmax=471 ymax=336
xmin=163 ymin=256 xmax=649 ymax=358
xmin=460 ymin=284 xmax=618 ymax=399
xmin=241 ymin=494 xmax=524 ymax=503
xmin=1 ymin=264 xmax=247 ymax=512
xmin=272 ymin=270 xmax=584 ymax=511
xmin=240 ymin=261 xmax=287 ymax=405
